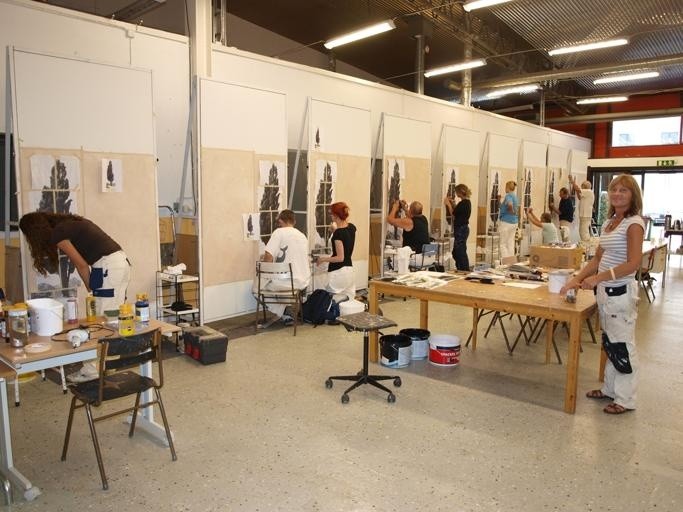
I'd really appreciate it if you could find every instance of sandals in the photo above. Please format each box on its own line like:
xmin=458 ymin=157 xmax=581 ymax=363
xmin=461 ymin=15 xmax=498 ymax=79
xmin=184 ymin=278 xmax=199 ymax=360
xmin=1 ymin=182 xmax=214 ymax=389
xmin=604 ymin=404 xmax=635 ymax=414
xmin=586 ymin=390 xmax=614 ymax=401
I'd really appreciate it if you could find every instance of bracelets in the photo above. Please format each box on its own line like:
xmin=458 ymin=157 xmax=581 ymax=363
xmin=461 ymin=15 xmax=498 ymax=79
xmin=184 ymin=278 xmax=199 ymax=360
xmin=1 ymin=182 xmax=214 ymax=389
xmin=609 ymin=267 xmax=616 ymax=281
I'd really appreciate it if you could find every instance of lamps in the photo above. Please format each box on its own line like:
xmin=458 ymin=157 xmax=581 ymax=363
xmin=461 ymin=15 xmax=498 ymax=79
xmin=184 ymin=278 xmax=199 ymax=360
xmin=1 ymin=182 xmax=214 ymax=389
xmin=324 ymin=19 xmax=396 ymax=50
xmin=424 ymin=59 xmax=487 ymax=79
xmin=548 ymin=38 xmax=628 ymax=57
xmin=576 ymin=96 xmax=628 ymax=104
xmin=593 ymin=72 xmax=659 ymax=85
xmin=463 ymin=0 xmax=512 ymax=12
xmin=486 ymin=83 xmax=541 ymax=102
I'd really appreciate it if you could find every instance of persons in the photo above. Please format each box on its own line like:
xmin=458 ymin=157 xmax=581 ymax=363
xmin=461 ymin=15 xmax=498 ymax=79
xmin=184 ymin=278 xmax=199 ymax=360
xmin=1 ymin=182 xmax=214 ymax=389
xmin=20 ymin=212 xmax=131 ymax=383
xmin=568 ymin=174 xmax=595 ymax=240
xmin=316 ymin=202 xmax=382 ymax=316
xmin=444 ymin=184 xmax=472 ymax=271
xmin=559 ymin=175 xmax=645 ymax=413
xmin=252 ymin=209 xmax=310 ymax=325
xmin=387 ymin=199 xmax=435 ymax=267
xmin=525 ymin=207 xmax=559 ymax=246
xmin=497 ymin=181 xmax=521 ymax=258
xmin=550 ymin=187 xmax=573 ymax=243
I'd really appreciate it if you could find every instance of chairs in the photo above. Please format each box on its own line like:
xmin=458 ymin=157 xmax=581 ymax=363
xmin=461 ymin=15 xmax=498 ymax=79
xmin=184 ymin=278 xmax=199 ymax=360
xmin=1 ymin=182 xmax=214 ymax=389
xmin=409 ymin=241 xmax=667 ymax=364
xmin=0 ymin=289 xmax=68 ymax=406
xmin=254 ymin=263 xmax=306 ymax=336
xmin=60 ymin=328 xmax=177 ymax=490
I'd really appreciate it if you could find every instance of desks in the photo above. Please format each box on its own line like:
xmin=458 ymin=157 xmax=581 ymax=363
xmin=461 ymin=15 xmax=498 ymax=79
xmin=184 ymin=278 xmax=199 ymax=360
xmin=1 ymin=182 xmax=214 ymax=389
xmin=369 ymin=271 xmax=608 ymax=414
xmin=0 ymin=316 xmax=180 ymax=501
xmin=666 ymin=229 xmax=683 ymax=259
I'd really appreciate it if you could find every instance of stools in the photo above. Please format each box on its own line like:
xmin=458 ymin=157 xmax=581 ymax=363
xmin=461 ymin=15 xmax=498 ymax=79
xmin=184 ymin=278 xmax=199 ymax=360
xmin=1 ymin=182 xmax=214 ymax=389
xmin=326 ymin=312 xmax=401 ymax=404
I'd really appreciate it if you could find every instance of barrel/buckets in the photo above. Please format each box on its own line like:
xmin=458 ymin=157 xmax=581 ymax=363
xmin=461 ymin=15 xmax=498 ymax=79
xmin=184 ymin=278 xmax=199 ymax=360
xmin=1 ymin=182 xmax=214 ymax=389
xmin=28 ymin=298 xmax=62 ymax=336
xmin=338 ymin=300 xmax=367 ymax=317
xmin=377 ymin=335 xmax=411 ymax=370
xmin=429 ymin=333 xmax=462 ymax=366
xmin=401 ymin=328 xmax=430 ymax=360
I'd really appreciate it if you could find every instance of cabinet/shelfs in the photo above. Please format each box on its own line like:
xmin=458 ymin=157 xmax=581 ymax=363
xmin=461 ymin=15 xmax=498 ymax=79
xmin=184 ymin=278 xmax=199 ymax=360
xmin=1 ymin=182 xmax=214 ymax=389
xmin=156 ymin=271 xmax=201 ymax=352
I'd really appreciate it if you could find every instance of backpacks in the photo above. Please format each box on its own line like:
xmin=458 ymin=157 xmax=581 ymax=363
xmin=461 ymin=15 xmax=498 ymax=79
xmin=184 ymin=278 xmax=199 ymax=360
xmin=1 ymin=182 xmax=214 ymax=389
xmin=299 ymin=289 xmax=333 ymax=325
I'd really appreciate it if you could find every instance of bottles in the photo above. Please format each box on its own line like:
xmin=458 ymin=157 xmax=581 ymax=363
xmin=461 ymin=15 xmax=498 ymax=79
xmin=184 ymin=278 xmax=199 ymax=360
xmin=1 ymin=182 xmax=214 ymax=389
xmin=67 ymin=298 xmax=78 ymax=323
xmin=567 ymin=287 xmax=576 ymax=304
xmin=135 ymin=293 xmax=150 ymax=325
xmin=118 ymin=304 xmax=135 ymax=336
xmin=0 ymin=301 xmax=32 ymax=348
xmin=86 ymin=292 xmax=96 ymax=321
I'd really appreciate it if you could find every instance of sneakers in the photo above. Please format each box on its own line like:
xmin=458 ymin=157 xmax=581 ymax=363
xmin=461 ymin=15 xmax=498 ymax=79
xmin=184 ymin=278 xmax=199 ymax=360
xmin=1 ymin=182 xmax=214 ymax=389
xmin=66 ymin=370 xmax=99 ymax=382
xmin=282 ymin=306 xmax=294 ymax=326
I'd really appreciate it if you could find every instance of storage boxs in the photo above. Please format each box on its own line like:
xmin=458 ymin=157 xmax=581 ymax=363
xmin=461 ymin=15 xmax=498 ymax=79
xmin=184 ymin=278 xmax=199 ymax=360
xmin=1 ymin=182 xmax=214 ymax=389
xmin=182 ymin=326 xmax=228 ymax=365
